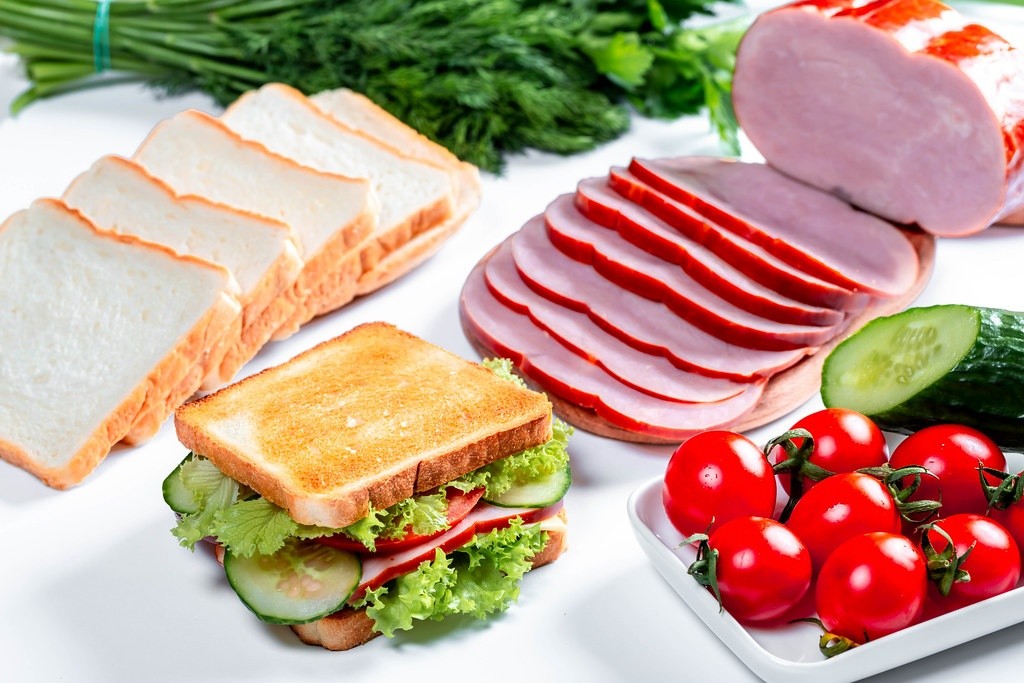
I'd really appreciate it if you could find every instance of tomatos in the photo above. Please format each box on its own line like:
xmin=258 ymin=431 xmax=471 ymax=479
xmin=667 ymin=408 xmax=1024 ymax=645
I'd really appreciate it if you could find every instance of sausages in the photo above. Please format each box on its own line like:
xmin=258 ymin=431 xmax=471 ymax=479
xmin=457 ymin=0 xmax=1024 ymax=445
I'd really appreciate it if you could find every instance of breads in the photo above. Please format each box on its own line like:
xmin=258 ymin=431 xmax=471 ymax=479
xmin=0 ymin=83 xmax=480 ymax=492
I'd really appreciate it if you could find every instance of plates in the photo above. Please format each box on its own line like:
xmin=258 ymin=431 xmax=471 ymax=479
xmin=627 ymin=423 xmax=1024 ymax=683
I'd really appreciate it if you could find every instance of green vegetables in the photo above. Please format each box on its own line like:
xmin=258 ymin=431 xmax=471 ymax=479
xmin=2 ymin=0 xmax=743 ymax=177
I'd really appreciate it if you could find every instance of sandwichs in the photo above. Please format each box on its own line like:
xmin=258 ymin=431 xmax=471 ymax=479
xmin=163 ymin=320 xmax=570 ymax=652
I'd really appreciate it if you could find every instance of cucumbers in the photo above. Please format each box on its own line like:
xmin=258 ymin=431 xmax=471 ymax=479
xmin=822 ymin=306 xmax=1024 ymax=450
